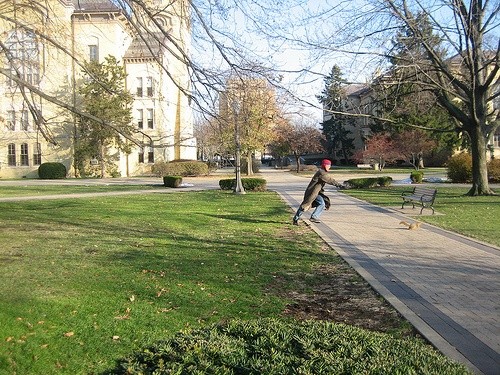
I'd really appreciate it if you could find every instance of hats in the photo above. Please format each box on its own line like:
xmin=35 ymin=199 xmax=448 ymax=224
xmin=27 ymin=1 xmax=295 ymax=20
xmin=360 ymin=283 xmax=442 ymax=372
xmin=322 ymin=160 xmax=331 ymax=167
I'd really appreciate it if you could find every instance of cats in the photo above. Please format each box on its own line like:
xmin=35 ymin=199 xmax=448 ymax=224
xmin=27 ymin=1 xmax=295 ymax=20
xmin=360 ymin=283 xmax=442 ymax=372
xmin=399 ymin=221 xmax=421 ymax=230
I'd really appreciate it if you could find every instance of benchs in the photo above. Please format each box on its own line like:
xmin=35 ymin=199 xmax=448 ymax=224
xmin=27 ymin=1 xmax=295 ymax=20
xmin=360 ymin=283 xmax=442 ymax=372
xmin=400 ymin=186 xmax=437 ymax=214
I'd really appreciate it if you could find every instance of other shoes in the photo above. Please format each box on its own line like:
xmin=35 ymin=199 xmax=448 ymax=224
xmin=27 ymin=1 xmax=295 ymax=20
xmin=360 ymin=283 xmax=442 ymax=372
xmin=293 ymin=219 xmax=298 ymax=226
xmin=310 ymin=217 xmax=321 ymax=223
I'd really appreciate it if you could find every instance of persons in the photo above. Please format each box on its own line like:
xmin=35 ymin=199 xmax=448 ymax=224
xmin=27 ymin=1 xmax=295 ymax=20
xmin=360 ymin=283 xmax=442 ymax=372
xmin=293 ymin=160 xmax=342 ymax=226
